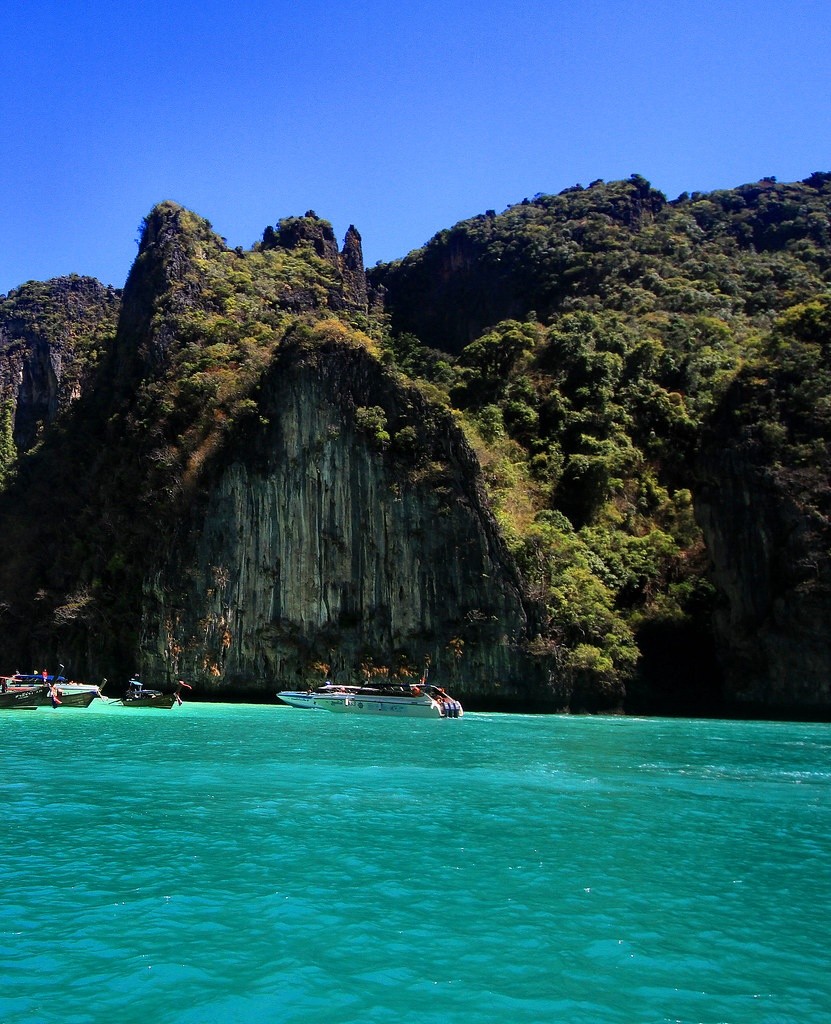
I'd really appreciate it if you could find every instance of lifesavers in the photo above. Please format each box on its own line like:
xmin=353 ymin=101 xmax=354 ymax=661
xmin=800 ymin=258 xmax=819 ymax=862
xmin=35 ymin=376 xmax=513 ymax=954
xmin=411 ymin=687 xmax=420 ymax=696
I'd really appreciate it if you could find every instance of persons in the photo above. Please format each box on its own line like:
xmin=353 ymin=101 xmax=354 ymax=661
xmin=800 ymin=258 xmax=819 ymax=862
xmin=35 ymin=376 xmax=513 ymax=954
xmin=68 ymin=680 xmax=84 ymax=686
xmin=42 ymin=668 xmax=49 ymax=685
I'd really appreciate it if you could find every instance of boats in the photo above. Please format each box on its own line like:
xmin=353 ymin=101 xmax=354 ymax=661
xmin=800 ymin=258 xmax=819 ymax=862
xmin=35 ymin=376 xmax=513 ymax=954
xmin=0 ymin=663 xmax=65 ymax=711
xmin=4 ymin=669 xmax=109 ymax=708
xmin=119 ymin=677 xmax=192 ymax=709
xmin=276 ymin=681 xmax=464 ymax=720
